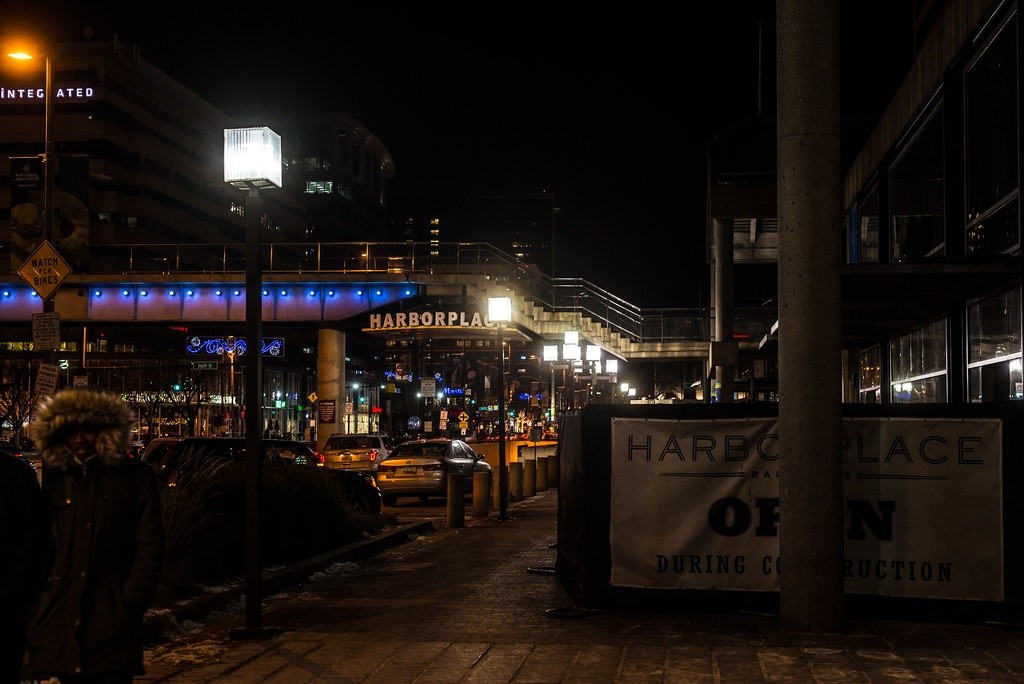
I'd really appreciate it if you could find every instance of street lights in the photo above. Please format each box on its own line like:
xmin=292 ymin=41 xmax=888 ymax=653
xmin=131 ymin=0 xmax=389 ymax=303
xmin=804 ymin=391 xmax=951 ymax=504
xmin=221 ymin=124 xmax=284 ymax=635
xmin=487 ymin=295 xmax=517 ymax=520
xmin=7 ymin=48 xmax=53 ymax=364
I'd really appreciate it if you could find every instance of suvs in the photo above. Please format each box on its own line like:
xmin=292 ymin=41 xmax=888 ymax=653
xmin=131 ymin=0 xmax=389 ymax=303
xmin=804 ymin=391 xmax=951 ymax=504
xmin=319 ymin=433 xmax=395 ymax=479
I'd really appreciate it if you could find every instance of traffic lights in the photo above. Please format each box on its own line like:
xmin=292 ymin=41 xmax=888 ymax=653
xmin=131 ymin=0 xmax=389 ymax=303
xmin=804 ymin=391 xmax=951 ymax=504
xmin=295 ymin=405 xmax=303 ymax=411
xmin=274 ymin=401 xmax=286 ymax=407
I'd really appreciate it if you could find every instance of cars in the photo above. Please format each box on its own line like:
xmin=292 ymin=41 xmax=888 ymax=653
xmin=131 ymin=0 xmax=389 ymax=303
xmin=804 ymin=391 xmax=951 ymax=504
xmin=142 ymin=436 xmax=382 ymax=564
xmin=270 ymin=440 xmax=325 ymax=467
xmin=375 ymin=439 xmax=492 ymax=507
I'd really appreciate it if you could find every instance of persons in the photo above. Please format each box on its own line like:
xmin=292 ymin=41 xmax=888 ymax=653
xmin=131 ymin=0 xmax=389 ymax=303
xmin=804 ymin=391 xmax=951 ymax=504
xmin=0 ymin=390 xmax=163 ymax=684
